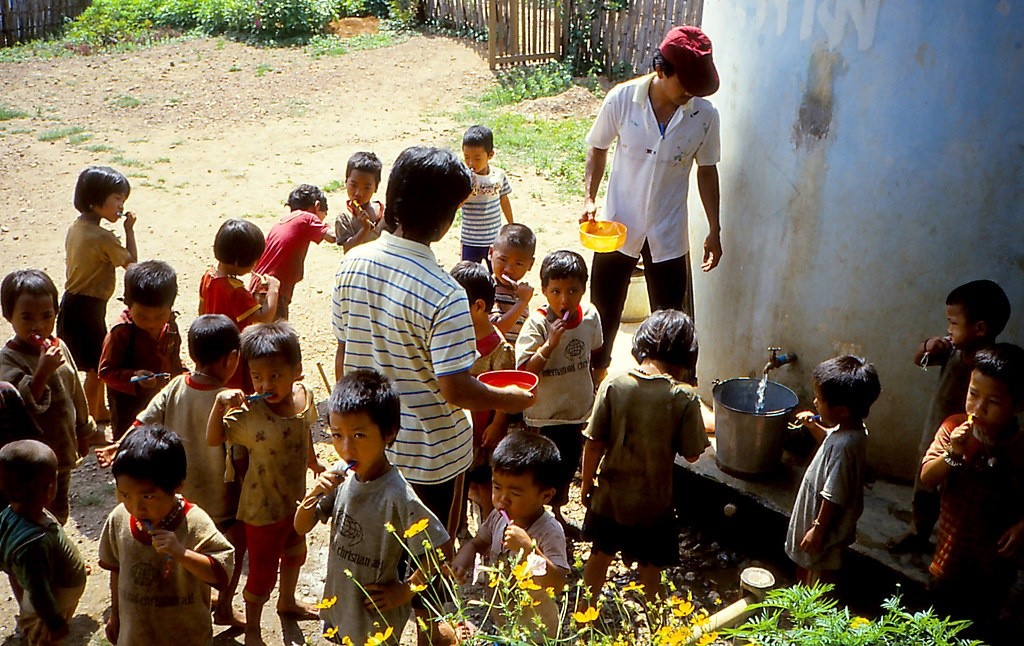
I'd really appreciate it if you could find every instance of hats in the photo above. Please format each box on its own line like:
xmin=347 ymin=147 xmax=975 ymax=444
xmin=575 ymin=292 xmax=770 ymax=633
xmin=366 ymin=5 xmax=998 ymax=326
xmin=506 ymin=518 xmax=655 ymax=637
xmin=659 ymin=26 xmax=720 ymax=98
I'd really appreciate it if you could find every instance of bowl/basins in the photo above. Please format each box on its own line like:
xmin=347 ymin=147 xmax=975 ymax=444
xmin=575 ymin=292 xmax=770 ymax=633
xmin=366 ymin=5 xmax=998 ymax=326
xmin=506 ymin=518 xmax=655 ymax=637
xmin=479 ymin=370 xmax=539 ymax=406
xmin=578 ymin=218 xmax=628 ymax=253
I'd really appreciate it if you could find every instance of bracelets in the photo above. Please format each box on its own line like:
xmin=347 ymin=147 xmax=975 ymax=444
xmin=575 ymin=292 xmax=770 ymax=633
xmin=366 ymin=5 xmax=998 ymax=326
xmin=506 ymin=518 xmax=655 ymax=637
xmin=812 ymin=519 xmax=828 ymax=529
xmin=536 ymin=347 xmax=548 ymax=361
xmin=923 ymin=338 xmax=932 ymax=353
xmin=943 ymin=447 xmax=964 ymax=467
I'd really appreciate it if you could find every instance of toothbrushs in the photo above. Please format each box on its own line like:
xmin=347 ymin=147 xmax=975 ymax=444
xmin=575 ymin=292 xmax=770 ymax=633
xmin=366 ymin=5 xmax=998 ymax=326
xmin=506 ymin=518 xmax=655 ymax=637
xmin=792 ymin=414 xmax=821 ymax=425
xmin=242 ymin=393 xmax=274 ymax=400
xmin=959 ymin=413 xmax=975 ymax=444
xmin=117 ymin=211 xmax=126 ymax=218
xmin=314 ymin=461 xmax=354 ymax=497
xmin=470 ymin=167 xmax=479 ymax=198
xmin=136 ymin=518 xmax=172 ymax=561
xmin=561 ymin=310 xmax=571 ymax=323
xmin=354 ymin=199 xmax=375 ymax=227
xmin=32 ymin=334 xmax=51 ymax=351
xmin=501 ymin=274 xmax=519 ymax=288
xmin=131 ymin=373 xmax=171 ymax=382
xmin=499 ymin=509 xmax=512 ymax=528
xmin=922 ymin=340 xmax=955 ymax=358
xmin=250 ymin=269 xmax=265 ymax=281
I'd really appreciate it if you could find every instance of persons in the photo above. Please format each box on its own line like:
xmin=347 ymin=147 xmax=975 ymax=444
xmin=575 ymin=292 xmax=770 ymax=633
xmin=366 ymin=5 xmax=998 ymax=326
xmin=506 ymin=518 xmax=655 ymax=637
xmin=460 ymin=125 xmax=514 ymax=275
xmin=440 ymin=431 xmax=571 ymax=646
xmin=575 ymin=308 xmax=710 ymax=632
xmin=1 ymin=269 xmax=97 ymax=577
xmin=451 ymin=261 xmax=515 ymax=560
xmin=784 ymin=354 xmax=882 ymax=601
xmin=93 ymin=314 xmax=246 ymax=627
xmin=205 ymin=321 xmax=327 ymax=645
xmin=487 ymin=223 xmax=536 ymax=348
xmin=884 ymin=280 xmax=1012 ymax=554
xmin=515 ymin=250 xmax=604 ymax=536
xmin=98 ymin=425 xmax=236 ymax=646
xmin=199 ymin=219 xmax=281 ymax=396
xmin=335 ymin=152 xmax=387 ymax=258
xmin=57 ymin=169 xmax=138 ymax=427
xmin=293 ymin=369 xmax=451 ymax=646
xmin=0 ymin=440 xmax=86 ymax=646
xmin=331 ymin=146 xmax=535 ymax=646
xmin=578 ymin=29 xmax=722 ymax=433
xmin=918 ymin=342 xmax=1023 ymax=646
xmin=249 ymin=184 xmax=336 ymax=322
xmin=0 ymin=381 xmax=45 ymax=608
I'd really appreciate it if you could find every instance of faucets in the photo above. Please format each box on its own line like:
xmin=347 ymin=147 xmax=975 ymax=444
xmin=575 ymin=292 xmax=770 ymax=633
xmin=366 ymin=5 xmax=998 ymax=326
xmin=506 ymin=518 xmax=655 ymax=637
xmin=762 ymin=346 xmax=798 ymax=376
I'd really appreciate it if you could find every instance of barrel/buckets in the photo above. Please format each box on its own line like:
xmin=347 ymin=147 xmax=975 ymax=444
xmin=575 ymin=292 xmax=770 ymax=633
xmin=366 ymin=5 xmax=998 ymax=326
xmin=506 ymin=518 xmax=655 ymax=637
xmin=707 ymin=372 xmax=800 ymax=481
